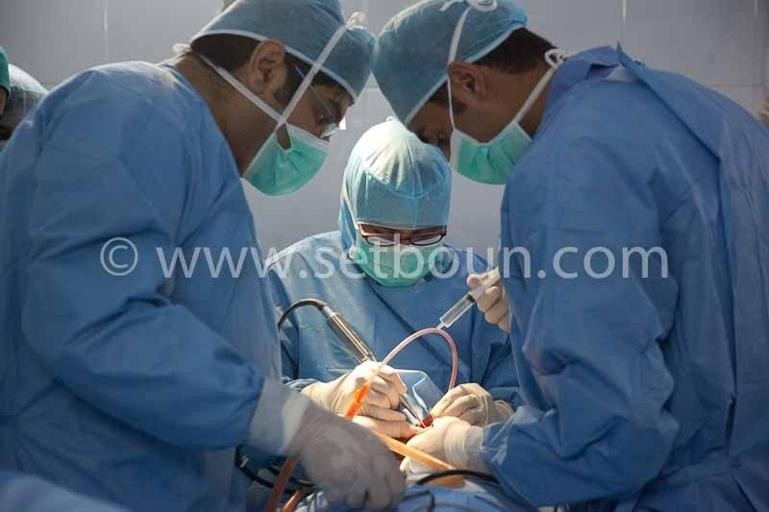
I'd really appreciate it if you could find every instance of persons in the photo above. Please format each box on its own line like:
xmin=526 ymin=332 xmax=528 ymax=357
xmin=399 ymin=1 xmax=768 ymax=512
xmin=0 ymin=45 xmax=12 ymax=120
xmin=0 ymin=63 xmax=47 ymax=151
xmin=0 ymin=0 xmax=408 ymax=511
xmin=247 ymin=115 xmax=531 ymax=439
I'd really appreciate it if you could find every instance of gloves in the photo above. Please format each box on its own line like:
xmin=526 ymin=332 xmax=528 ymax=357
xmin=248 ymin=376 xmax=405 ymax=510
xmin=302 ymin=360 xmax=514 ymax=473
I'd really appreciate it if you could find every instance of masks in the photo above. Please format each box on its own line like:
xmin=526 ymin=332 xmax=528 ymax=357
xmin=449 ymin=122 xmax=534 ymax=185
xmin=243 ymin=122 xmax=329 ymax=197
xmin=347 ymin=229 xmax=444 ymax=288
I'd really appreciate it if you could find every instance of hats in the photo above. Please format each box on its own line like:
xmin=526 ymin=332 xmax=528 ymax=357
xmin=190 ymin=0 xmax=375 ymax=107
xmin=339 ymin=118 xmax=451 ymax=251
xmin=372 ymin=0 xmax=526 ymax=132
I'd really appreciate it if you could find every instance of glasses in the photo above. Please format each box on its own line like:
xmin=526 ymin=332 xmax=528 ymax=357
xmin=292 ymin=61 xmax=347 ymax=139
xmin=359 ymin=227 xmax=447 ymax=246
